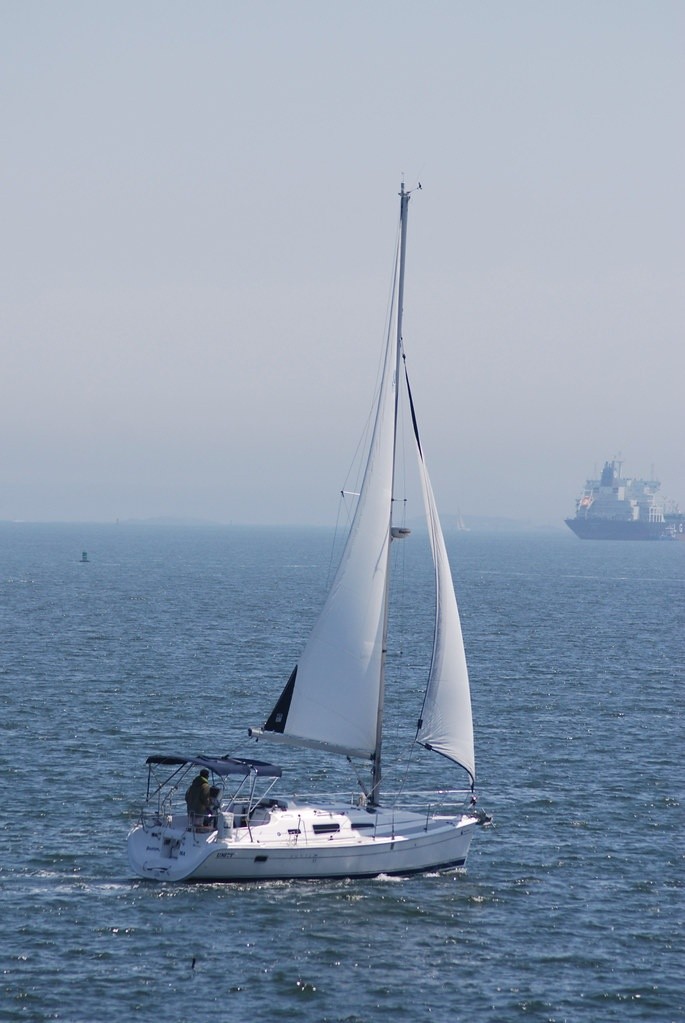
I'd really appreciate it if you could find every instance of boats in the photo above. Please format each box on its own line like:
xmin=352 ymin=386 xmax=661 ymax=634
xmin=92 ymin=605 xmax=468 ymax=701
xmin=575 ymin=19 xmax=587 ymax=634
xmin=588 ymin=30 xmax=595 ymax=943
xmin=562 ymin=451 xmax=685 ymax=541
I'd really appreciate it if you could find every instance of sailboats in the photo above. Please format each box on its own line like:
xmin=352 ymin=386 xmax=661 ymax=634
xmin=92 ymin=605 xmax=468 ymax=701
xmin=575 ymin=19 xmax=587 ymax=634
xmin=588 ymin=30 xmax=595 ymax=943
xmin=123 ymin=167 xmax=497 ymax=885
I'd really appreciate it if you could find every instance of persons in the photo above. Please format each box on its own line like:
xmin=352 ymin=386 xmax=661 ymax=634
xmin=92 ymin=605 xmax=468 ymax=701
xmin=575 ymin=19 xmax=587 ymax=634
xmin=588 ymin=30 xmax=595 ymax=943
xmin=185 ymin=769 xmax=211 ymax=818
